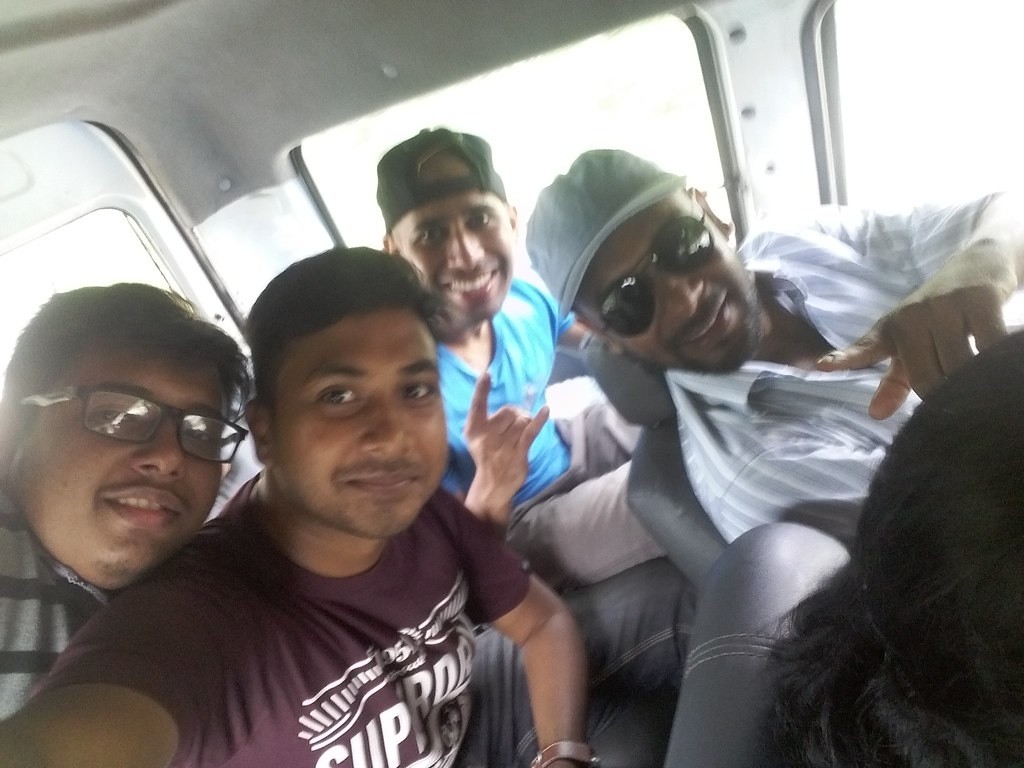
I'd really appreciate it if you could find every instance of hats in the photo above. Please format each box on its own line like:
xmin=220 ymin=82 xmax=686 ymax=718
xmin=525 ymin=148 xmax=688 ymax=320
xmin=376 ymin=128 xmax=508 ymax=235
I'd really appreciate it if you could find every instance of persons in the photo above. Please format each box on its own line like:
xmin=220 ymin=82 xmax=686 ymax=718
xmin=520 ymin=147 xmax=1023 ymax=550
xmin=0 ymin=244 xmax=698 ymax=768
xmin=0 ymin=282 xmax=252 ymax=726
xmin=770 ymin=327 xmax=1023 ymax=768
xmin=379 ymin=126 xmax=669 ymax=589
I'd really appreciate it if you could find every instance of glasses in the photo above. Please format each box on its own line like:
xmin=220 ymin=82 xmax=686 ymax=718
xmin=21 ymin=383 xmax=248 ymax=463
xmin=599 ymin=210 xmax=712 ymax=340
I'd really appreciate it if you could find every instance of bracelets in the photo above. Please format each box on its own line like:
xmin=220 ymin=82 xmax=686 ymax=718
xmin=529 ymin=740 xmax=599 ymax=767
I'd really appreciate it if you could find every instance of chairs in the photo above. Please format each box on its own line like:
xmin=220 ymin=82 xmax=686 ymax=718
xmin=670 ymin=525 xmax=854 ymax=768
xmin=585 ymin=332 xmax=725 ymax=585
